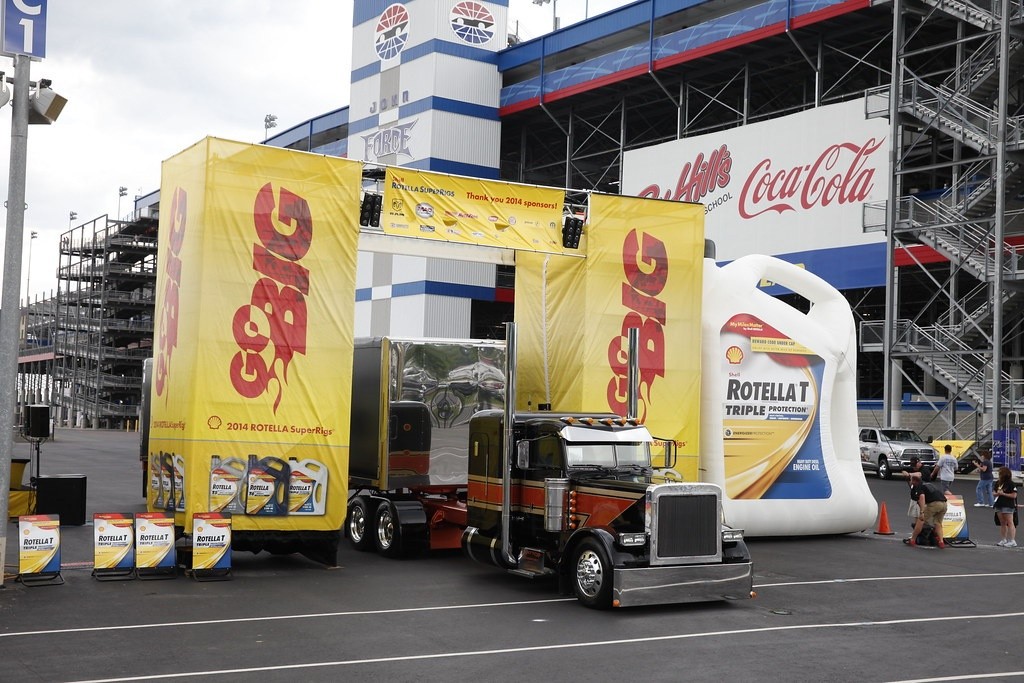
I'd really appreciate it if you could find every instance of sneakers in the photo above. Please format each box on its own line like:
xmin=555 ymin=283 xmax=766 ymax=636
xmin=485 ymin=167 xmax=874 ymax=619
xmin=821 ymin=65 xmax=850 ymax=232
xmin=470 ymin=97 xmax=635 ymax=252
xmin=1003 ymin=540 xmax=1017 ymax=547
xmin=997 ymin=539 xmax=1007 ymax=546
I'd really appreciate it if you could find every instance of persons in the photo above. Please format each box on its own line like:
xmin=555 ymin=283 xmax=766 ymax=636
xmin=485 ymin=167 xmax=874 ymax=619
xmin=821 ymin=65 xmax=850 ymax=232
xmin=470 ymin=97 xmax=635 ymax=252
xmin=930 ymin=445 xmax=958 ymax=493
xmin=974 ymin=451 xmax=994 ymax=508
xmin=902 ymin=456 xmax=932 ymax=529
xmin=993 ymin=466 xmax=1018 ymax=548
xmin=903 ymin=482 xmax=947 ymax=549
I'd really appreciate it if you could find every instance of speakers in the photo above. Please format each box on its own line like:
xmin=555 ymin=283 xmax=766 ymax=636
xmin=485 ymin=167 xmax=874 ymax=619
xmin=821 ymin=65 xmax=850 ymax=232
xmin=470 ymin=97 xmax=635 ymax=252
xmin=27 ymin=87 xmax=68 ymax=125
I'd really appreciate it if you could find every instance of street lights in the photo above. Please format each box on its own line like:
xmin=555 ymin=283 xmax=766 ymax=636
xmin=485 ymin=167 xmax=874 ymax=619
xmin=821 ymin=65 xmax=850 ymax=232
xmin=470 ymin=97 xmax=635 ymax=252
xmin=0 ymin=74 xmax=67 ymax=589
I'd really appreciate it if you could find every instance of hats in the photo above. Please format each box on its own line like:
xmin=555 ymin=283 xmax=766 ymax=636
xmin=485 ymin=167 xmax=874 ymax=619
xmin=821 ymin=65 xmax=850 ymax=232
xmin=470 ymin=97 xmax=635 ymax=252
xmin=909 ymin=457 xmax=920 ymax=465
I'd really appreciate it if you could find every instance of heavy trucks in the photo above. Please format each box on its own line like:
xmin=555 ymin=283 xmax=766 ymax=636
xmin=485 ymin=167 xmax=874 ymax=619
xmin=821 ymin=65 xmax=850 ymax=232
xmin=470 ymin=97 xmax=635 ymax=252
xmin=352 ymin=333 xmax=756 ymax=611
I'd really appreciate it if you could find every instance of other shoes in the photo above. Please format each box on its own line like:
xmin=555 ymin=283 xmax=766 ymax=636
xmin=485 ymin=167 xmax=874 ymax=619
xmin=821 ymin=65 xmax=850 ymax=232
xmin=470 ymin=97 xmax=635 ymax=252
xmin=903 ymin=538 xmax=916 ymax=547
xmin=935 ymin=536 xmax=944 ymax=548
xmin=974 ymin=503 xmax=984 ymax=506
xmin=985 ymin=504 xmax=993 ymax=507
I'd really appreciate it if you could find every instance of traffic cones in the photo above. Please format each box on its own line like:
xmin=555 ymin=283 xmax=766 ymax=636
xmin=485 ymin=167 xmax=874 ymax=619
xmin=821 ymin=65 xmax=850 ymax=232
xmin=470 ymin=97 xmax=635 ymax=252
xmin=874 ymin=502 xmax=896 ymax=537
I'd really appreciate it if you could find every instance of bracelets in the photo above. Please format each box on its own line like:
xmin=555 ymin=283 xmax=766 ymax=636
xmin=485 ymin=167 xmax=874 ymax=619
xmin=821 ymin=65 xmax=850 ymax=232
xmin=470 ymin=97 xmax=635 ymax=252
xmin=909 ymin=473 xmax=911 ymax=477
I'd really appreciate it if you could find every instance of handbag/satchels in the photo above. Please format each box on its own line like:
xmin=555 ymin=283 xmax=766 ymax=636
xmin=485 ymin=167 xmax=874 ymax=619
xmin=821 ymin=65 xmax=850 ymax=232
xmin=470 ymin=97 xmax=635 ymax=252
xmin=994 ymin=510 xmax=1018 ymax=526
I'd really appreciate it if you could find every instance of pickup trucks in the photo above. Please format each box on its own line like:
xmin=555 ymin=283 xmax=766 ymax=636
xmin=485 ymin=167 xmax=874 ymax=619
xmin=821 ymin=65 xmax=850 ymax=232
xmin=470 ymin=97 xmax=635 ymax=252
xmin=858 ymin=426 xmax=939 ymax=481
